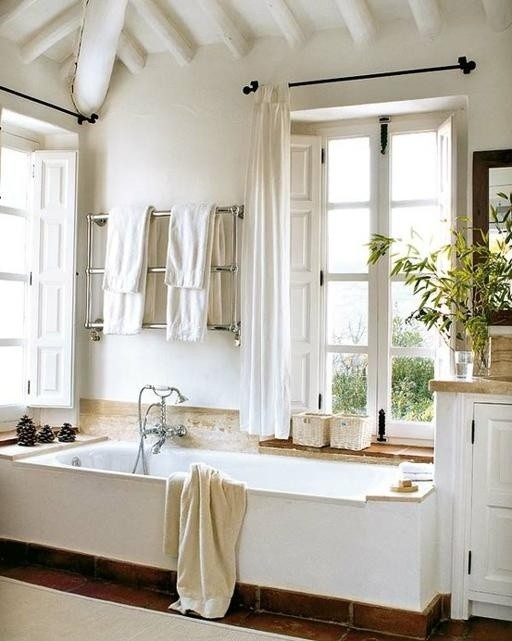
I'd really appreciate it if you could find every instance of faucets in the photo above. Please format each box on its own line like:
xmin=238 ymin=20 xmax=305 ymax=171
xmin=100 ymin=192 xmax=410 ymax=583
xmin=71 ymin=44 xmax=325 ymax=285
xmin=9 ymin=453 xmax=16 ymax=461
xmin=149 ymin=427 xmax=174 ymax=456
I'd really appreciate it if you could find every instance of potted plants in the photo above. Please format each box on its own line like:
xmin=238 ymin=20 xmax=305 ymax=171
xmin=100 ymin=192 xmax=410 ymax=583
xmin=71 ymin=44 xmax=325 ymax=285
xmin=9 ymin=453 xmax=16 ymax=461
xmin=364 ymin=205 xmax=512 ymax=380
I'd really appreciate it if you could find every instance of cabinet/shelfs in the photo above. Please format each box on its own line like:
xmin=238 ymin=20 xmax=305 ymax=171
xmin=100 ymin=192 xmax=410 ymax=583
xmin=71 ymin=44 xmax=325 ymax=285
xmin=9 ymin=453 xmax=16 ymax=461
xmin=450 ymin=394 xmax=511 ymax=623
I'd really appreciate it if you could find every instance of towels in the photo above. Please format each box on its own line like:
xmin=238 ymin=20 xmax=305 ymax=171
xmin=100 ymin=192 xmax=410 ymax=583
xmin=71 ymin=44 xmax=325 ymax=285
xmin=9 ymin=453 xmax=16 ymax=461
xmin=164 ymin=201 xmax=229 ymax=343
xmin=104 ymin=204 xmax=157 ymax=339
xmin=164 ymin=463 xmax=248 ymax=623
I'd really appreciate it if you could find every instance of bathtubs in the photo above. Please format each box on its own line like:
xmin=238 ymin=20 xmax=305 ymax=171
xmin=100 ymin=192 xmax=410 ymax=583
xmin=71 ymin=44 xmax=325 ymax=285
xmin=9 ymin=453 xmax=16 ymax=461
xmin=0 ymin=429 xmax=435 ymax=640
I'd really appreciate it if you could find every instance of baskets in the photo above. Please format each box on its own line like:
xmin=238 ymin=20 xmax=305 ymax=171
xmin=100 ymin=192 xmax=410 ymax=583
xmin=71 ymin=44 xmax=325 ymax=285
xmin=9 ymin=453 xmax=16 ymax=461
xmin=330 ymin=414 xmax=371 ymax=451
xmin=292 ymin=413 xmax=334 ymax=448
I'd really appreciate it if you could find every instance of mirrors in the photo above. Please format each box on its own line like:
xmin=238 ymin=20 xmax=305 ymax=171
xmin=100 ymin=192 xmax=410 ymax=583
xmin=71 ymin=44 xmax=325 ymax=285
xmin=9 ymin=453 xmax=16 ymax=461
xmin=471 ymin=150 xmax=512 ymax=324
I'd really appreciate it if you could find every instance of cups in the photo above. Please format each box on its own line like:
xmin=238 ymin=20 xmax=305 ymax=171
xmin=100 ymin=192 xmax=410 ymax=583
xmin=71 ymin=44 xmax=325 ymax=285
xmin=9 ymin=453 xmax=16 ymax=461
xmin=454 ymin=351 xmax=474 ymax=379
